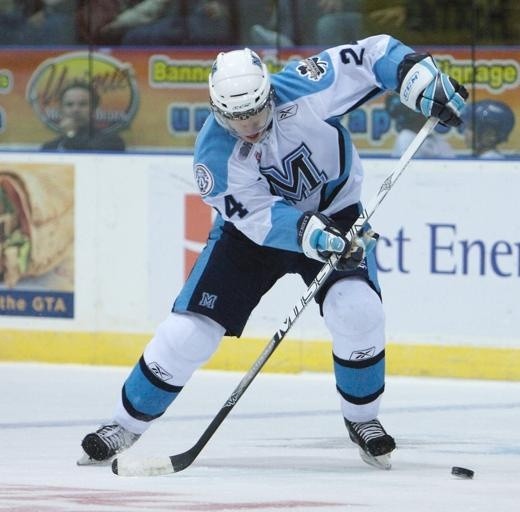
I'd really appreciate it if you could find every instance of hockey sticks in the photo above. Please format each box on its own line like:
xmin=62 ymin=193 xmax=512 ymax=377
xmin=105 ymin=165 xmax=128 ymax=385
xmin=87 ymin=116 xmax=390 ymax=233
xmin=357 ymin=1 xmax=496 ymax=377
xmin=112 ymin=115 xmax=440 ymax=475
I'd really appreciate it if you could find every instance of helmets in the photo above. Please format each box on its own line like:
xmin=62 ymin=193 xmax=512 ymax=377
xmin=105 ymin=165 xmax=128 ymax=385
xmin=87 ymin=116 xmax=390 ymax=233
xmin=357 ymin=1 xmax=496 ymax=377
xmin=207 ymin=47 xmax=271 ymax=121
xmin=456 ymin=99 xmax=514 ymax=142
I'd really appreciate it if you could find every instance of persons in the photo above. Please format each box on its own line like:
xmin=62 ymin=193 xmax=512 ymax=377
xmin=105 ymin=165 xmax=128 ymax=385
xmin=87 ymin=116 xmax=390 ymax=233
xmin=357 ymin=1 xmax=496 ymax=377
xmin=80 ymin=32 xmax=469 ymax=457
xmin=39 ymin=83 xmax=123 ymax=153
xmin=386 ymin=90 xmax=455 ymax=157
xmin=0 ymin=1 xmax=520 ymax=52
xmin=457 ymin=98 xmax=512 ymax=158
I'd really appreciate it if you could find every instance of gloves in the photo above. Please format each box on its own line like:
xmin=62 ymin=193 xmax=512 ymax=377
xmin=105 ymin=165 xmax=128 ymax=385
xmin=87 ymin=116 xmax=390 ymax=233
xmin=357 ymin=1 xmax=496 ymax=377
xmin=296 ymin=210 xmax=366 ymax=272
xmin=394 ymin=50 xmax=469 ymax=129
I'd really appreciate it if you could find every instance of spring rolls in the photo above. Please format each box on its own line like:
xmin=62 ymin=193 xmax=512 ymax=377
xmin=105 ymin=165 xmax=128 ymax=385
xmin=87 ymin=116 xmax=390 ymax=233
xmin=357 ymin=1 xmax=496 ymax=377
xmin=0 ymin=163 xmax=74 ymax=287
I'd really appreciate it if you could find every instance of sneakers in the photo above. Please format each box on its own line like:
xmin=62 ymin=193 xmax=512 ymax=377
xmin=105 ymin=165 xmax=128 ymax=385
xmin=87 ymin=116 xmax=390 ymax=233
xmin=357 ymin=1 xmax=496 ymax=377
xmin=344 ymin=416 xmax=397 ymax=458
xmin=82 ymin=421 xmax=141 ymax=462
